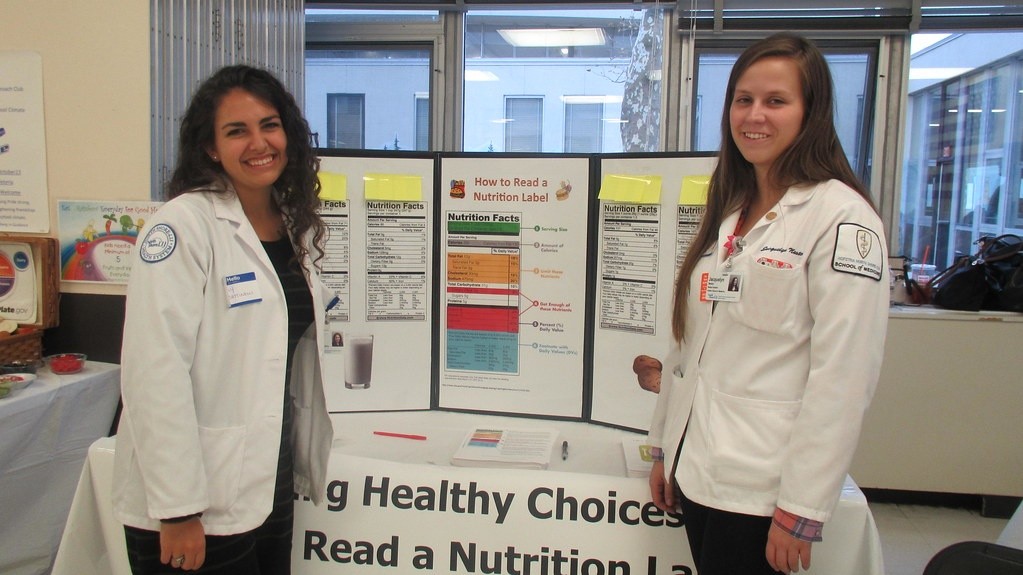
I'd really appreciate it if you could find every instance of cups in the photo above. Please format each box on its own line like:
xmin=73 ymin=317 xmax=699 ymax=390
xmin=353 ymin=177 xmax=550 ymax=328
xmin=911 ymin=264 xmax=936 ymax=303
xmin=344 ymin=333 xmax=374 ymax=390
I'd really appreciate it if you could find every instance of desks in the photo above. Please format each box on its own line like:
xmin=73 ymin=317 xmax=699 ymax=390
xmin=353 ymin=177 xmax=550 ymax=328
xmin=0 ymin=356 xmax=123 ymax=575
xmin=51 ymin=406 xmax=886 ymax=575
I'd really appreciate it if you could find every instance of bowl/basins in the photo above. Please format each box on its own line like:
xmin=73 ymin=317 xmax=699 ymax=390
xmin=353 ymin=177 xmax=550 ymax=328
xmin=41 ymin=352 xmax=88 ymax=375
xmin=0 ymin=359 xmax=40 ymax=375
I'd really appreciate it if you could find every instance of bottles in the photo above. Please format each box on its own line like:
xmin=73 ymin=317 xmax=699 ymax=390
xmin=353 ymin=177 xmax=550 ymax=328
xmin=888 ymin=265 xmax=895 ymax=307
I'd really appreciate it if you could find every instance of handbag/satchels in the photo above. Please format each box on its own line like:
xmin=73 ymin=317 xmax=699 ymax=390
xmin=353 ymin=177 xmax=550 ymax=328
xmin=888 ymin=255 xmax=924 ymax=306
xmin=926 ymin=234 xmax=1023 ymax=313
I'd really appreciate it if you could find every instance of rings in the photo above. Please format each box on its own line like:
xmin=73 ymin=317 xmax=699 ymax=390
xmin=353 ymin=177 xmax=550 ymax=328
xmin=176 ymin=555 xmax=185 ymax=565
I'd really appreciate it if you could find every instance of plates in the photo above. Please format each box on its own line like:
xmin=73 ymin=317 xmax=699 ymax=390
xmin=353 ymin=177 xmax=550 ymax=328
xmin=0 ymin=370 xmax=38 ymax=390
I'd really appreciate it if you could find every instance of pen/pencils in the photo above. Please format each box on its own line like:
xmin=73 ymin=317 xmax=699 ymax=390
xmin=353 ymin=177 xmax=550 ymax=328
xmin=373 ymin=431 xmax=426 ymax=441
xmin=562 ymin=440 xmax=568 ymax=460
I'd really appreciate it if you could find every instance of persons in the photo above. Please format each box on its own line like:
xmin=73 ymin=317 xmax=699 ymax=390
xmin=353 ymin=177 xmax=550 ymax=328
xmin=332 ymin=333 xmax=343 ymax=347
xmin=728 ymin=277 xmax=739 ymax=291
xmin=645 ymin=31 xmax=890 ymax=575
xmin=112 ymin=64 xmax=338 ymax=575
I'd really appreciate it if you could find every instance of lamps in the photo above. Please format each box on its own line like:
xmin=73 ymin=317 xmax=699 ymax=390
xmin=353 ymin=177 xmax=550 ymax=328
xmin=495 ymin=26 xmax=610 ymax=48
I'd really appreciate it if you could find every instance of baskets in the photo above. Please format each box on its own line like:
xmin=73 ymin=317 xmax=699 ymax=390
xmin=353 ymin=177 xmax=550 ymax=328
xmin=0 ymin=329 xmax=43 ymax=367
